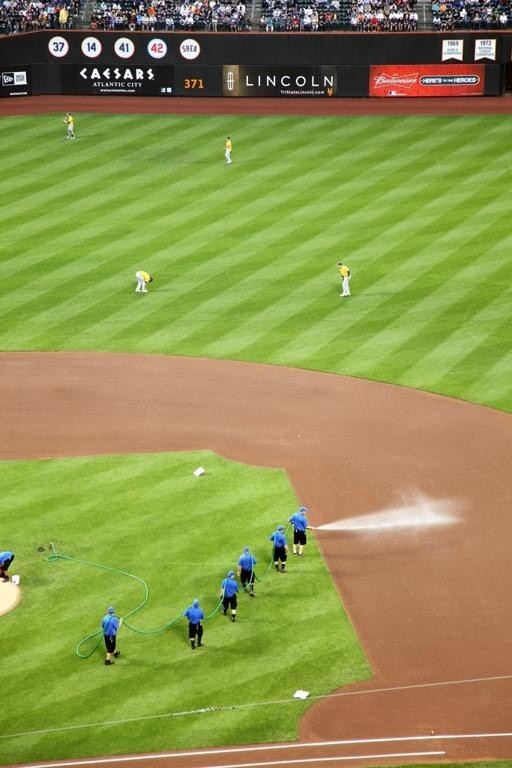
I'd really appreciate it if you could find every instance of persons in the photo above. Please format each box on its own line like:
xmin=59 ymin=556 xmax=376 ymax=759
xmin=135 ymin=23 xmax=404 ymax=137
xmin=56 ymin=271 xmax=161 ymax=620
xmin=0 ymin=551 xmax=14 ymax=580
xmin=63 ymin=111 xmax=75 ymax=139
xmin=270 ymin=525 xmax=289 ymax=573
xmin=219 ymin=570 xmax=239 ymax=622
xmin=135 ymin=270 xmax=153 ymax=293
xmin=337 ymin=262 xmax=352 ymax=297
xmin=183 ymin=600 xmax=204 ymax=650
xmin=288 ymin=506 xmax=309 ymax=558
xmin=237 ymin=546 xmax=257 ymax=596
xmin=224 ymin=136 xmax=232 ymax=164
xmin=102 ymin=607 xmax=123 ymax=665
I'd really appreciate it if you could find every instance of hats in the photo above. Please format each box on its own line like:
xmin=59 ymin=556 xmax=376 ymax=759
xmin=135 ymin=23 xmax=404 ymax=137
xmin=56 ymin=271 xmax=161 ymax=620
xmin=228 ymin=571 xmax=235 ymax=576
xmin=194 ymin=600 xmax=199 ymax=607
xmin=244 ymin=547 xmax=249 ymax=552
xmin=109 ymin=607 xmax=113 ymax=612
xmin=278 ymin=526 xmax=285 ymax=531
xmin=300 ymin=507 xmax=307 ymax=511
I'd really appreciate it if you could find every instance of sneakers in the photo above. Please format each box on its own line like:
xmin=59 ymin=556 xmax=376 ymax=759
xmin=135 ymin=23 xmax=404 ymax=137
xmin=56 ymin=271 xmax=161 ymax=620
xmin=198 ymin=644 xmax=202 ymax=647
xmin=105 ymin=661 xmax=113 ymax=665
xmin=0 ymin=573 xmax=4 ymax=577
xmin=3 ymin=578 xmax=9 ymax=582
xmin=115 ymin=651 xmax=120 ymax=658
xmin=192 ymin=646 xmax=196 ymax=649
